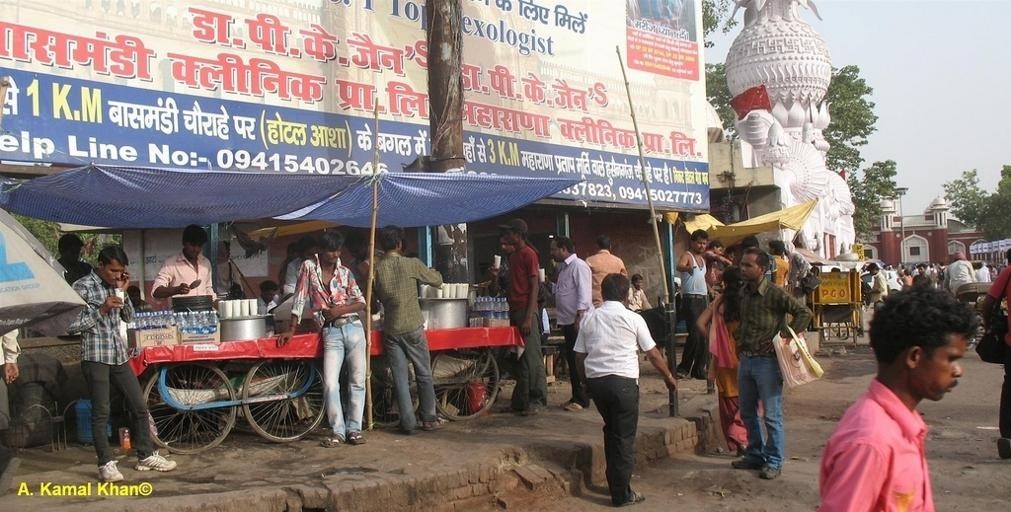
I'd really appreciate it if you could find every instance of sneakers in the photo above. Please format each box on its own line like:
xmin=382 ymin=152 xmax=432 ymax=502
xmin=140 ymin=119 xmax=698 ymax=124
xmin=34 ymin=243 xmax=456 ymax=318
xmin=97 ymin=458 xmax=124 ymax=483
xmin=423 ymin=416 xmax=449 ymax=431
xmin=732 ymin=455 xmax=759 ymax=471
xmin=521 ymin=403 xmax=548 ymax=416
xmin=400 ymin=422 xmax=419 ymax=434
xmin=758 ymin=462 xmax=783 ymax=480
xmin=622 ymin=491 xmax=646 ymax=506
xmin=561 ymin=400 xmax=574 ymax=408
xmin=997 ymin=436 xmax=1011 ymax=459
xmin=136 ymin=454 xmax=177 ymax=473
xmin=565 ymin=402 xmax=589 ymax=411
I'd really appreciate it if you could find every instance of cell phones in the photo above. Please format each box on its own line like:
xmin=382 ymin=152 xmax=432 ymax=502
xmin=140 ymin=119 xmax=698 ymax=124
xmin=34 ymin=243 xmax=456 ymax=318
xmin=122 ymin=273 xmax=126 ymax=280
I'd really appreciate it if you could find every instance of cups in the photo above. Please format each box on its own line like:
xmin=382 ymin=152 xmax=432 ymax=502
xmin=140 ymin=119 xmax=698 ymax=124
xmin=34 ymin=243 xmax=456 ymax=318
xmin=218 ymin=298 xmax=259 ymax=319
xmin=539 ymin=268 xmax=545 ymax=283
xmin=494 ymin=255 xmax=502 ymax=269
xmin=114 ymin=288 xmax=125 ymax=308
xmin=419 ymin=282 xmax=468 ymax=299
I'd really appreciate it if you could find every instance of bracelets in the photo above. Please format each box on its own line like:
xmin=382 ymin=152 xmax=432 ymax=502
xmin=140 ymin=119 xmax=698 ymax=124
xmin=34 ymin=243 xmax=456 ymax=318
xmin=289 ymin=325 xmax=297 ymax=329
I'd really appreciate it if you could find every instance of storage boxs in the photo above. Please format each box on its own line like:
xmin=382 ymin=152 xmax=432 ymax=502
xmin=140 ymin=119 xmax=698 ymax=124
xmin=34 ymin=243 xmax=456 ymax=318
xmin=179 ymin=323 xmax=220 ymax=346
xmin=127 ymin=325 xmax=179 ymax=347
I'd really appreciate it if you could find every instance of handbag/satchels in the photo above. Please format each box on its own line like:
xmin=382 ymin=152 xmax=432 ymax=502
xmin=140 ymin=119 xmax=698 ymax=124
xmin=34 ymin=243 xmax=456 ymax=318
xmin=976 ymin=326 xmax=1006 ymax=365
xmin=799 ymin=272 xmax=822 ymax=293
xmin=771 ymin=326 xmax=826 ymax=389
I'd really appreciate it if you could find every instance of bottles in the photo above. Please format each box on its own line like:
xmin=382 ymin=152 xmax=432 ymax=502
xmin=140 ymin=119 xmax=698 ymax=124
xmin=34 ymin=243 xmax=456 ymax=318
xmin=128 ymin=310 xmax=218 ymax=334
xmin=469 ymin=297 xmax=509 ymax=329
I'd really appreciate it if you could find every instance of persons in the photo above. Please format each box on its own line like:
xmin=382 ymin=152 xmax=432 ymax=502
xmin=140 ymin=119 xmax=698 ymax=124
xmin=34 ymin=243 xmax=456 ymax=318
xmin=483 ymin=218 xmax=814 ymax=505
xmin=372 ymin=224 xmax=442 ymax=433
xmin=864 ymin=250 xmax=1010 ymax=342
xmin=278 ymin=229 xmax=367 ymax=448
xmin=127 ymin=286 xmax=147 ymax=307
xmin=150 ymin=225 xmax=222 ymax=311
xmin=64 ymin=246 xmax=176 ymax=483
xmin=0 ymin=329 xmax=22 ymax=495
xmin=258 ymin=282 xmax=278 ymax=315
xmin=56 ymin=234 xmax=92 ymax=286
xmin=283 ymin=236 xmax=315 ymax=295
xmin=343 ymin=231 xmax=384 ymax=291
xmin=818 ymin=285 xmax=980 ymax=512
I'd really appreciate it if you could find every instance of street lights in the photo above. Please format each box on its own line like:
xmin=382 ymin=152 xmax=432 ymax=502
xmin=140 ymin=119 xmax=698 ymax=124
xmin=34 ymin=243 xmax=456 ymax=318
xmin=892 ymin=188 xmax=909 ymax=263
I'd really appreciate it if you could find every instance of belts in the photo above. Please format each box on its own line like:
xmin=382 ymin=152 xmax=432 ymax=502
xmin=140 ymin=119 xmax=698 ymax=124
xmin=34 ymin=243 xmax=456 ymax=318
xmin=682 ymin=293 xmax=708 ymax=301
xmin=738 ymin=350 xmax=775 ymax=358
xmin=320 ymin=314 xmax=362 ymax=327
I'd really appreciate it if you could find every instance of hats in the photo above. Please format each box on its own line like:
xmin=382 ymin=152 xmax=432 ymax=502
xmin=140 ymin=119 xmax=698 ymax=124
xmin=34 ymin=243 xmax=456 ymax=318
xmin=953 ymin=249 xmax=965 ymax=259
xmin=497 ymin=218 xmax=529 ymax=235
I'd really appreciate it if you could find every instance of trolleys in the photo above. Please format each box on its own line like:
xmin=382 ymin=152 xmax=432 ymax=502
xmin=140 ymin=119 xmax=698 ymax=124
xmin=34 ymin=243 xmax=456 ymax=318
xmin=113 ymin=333 xmax=325 ymax=456
xmin=355 ymin=327 xmax=526 ymax=425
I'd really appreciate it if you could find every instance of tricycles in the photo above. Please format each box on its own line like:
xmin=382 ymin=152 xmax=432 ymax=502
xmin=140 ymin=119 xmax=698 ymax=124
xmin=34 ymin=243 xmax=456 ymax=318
xmin=955 ymin=282 xmax=993 ymax=351
xmin=809 ymin=258 xmax=864 ymax=346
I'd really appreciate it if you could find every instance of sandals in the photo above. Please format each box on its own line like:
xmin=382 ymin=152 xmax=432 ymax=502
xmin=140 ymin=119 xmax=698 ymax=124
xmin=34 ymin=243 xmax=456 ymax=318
xmin=345 ymin=432 xmax=367 ymax=447
xmin=321 ymin=432 xmax=346 ymax=449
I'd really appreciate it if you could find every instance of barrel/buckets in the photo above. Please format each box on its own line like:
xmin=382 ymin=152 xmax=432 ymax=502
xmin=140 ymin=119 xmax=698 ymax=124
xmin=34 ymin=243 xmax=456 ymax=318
xmin=220 ymin=313 xmax=274 ymax=342
xmin=419 ymin=297 xmax=472 ymax=330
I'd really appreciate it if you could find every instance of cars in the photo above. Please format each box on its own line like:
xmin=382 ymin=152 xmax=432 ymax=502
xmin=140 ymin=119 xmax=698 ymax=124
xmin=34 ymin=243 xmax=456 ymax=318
xmin=859 ymin=271 xmax=903 ymax=293
